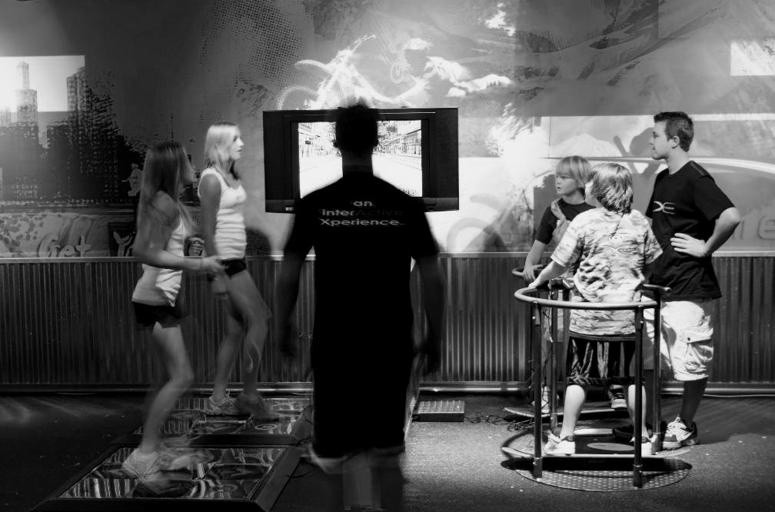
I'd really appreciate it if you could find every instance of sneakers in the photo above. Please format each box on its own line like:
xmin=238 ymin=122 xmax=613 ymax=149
xmin=544 ymin=433 xmax=576 ymax=455
xmin=156 ymin=445 xmax=191 ymax=470
xmin=233 ymin=391 xmax=280 ymax=421
xmin=205 ymin=393 xmax=242 ymax=415
xmin=629 ymin=415 xmax=700 ymax=450
xmin=608 ymin=383 xmax=628 ymax=408
xmin=531 ymin=385 xmax=560 ymax=414
xmin=121 ymin=448 xmax=170 ymax=494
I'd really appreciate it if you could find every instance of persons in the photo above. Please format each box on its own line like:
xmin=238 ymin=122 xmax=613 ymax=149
xmin=117 ymin=138 xmax=230 ymax=485
xmin=607 ymin=109 xmax=745 ymax=451
xmin=521 ymin=155 xmax=627 ymax=421
xmin=523 ymin=161 xmax=661 ymax=460
xmin=267 ymin=102 xmax=451 ymax=512
xmin=196 ymin=120 xmax=282 ymax=424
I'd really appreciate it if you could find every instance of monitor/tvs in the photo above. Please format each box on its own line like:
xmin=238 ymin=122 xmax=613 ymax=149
xmin=262 ymin=108 xmax=459 ymax=213
